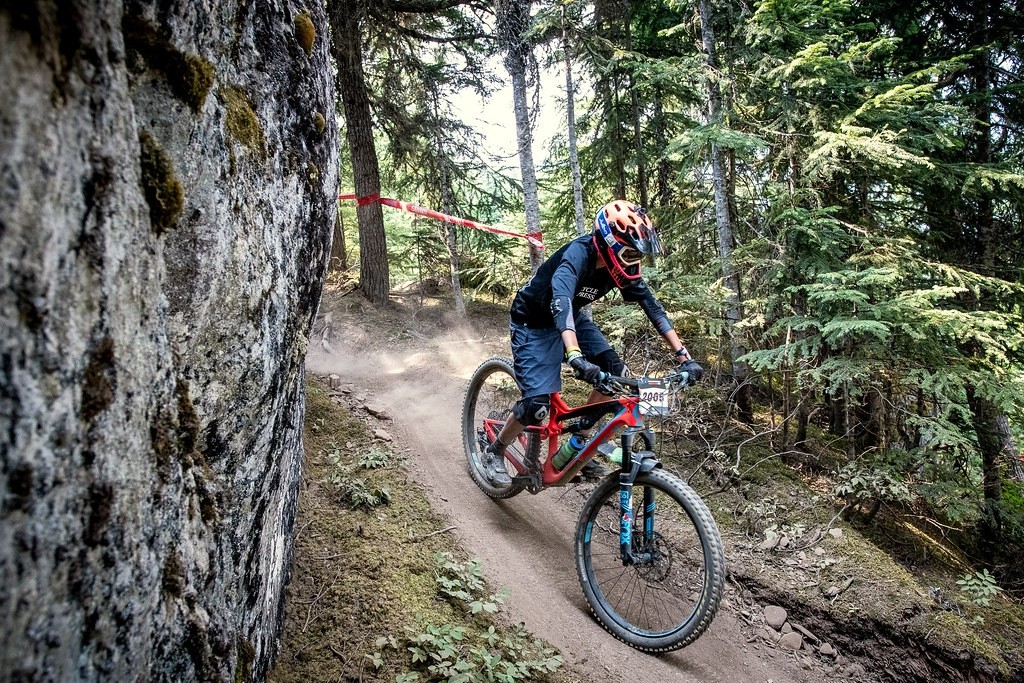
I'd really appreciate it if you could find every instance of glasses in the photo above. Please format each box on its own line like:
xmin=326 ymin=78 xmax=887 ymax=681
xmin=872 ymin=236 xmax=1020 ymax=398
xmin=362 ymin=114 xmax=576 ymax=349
xmin=597 ymin=207 xmax=646 ymax=265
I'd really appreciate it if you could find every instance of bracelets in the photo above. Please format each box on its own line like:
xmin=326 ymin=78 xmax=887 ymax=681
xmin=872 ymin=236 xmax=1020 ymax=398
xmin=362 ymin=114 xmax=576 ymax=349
xmin=675 ymin=346 xmax=686 ymax=356
xmin=566 ymin=346 xmax=584 ymax=364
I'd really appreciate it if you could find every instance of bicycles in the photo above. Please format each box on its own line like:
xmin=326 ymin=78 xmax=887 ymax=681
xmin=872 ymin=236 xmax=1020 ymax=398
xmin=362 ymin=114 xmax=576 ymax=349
xmin=460 ymin=345 xmax=727 ymax=654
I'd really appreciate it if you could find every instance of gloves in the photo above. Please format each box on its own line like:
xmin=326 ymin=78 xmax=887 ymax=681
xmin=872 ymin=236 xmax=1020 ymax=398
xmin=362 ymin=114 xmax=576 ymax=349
xmin=565 ymin=346 xmax=601 ymax=387
xmin=677 ymin=346 xmax=703 ymax=386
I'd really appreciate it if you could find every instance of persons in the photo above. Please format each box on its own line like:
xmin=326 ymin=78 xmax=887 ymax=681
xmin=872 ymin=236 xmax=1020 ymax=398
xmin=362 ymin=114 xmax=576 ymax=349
xmin=480 ymin=200 xmax=703 ymax=489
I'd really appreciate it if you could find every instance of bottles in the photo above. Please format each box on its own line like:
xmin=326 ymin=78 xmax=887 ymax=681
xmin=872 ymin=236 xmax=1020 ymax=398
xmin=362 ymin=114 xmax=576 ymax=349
xmin=551 ymin=436 xmax=584 ymax=471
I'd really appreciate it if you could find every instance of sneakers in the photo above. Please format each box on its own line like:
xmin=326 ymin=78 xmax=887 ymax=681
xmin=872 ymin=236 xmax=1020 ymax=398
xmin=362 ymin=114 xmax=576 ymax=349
xmin=580 ymin=459 xmax=604 ymax=478
xmin=481 ymin=445 xmax=512 ymax=488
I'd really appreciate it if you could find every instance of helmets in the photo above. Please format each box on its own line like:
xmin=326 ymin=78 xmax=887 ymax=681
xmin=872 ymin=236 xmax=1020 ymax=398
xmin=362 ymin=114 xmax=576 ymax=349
xmin=592 ymin=200 xmax=662 ymax=289
